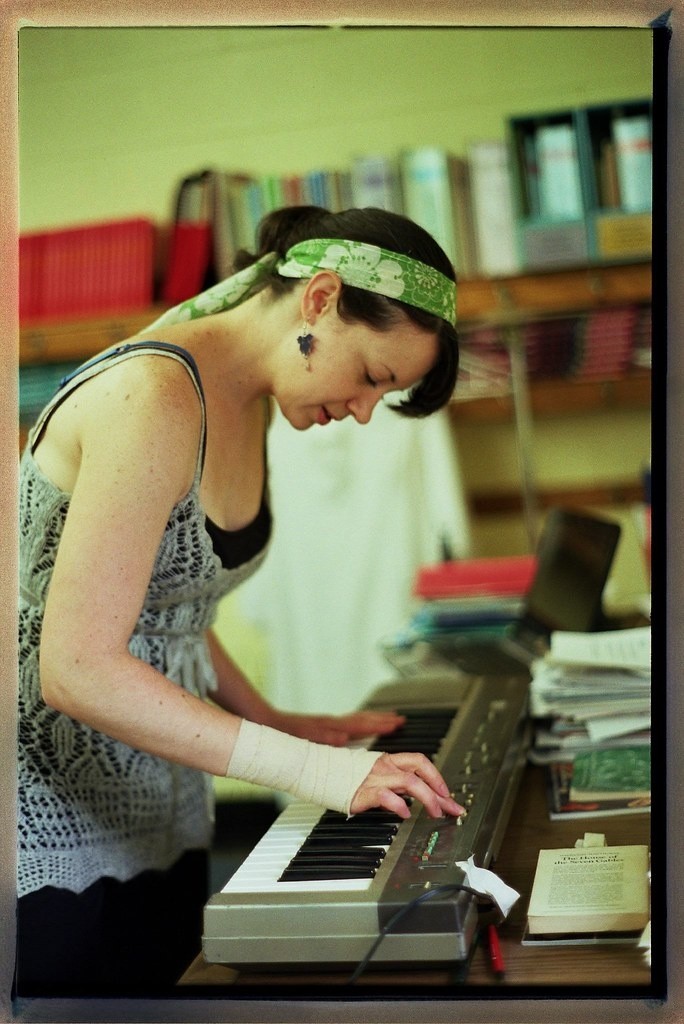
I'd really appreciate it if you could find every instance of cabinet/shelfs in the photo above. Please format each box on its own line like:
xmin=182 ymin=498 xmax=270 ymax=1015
xmin=19 ymin=260 xmax=651 ymax=520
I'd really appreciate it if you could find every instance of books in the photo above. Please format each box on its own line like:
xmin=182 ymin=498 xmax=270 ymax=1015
xmin=199 ymin=143 xmax=520 ymax=284
xmin=519 ymin=113 xmax=583 ymax=272
xmin=604 ymin=115 xmax=653 ymax=266
xmin=394 ymin=556 xmax=535 ymax=648
xmin=523 ymin=622 xmax=651 ymax=819
xmin=520 ymin=829 xmax=649 ymax=949
xmin=17 ymin=220 xmax=208 ymax=326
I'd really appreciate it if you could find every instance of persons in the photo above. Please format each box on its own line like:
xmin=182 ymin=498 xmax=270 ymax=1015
xmin=16 ymin=205 xmax=466 ymax=998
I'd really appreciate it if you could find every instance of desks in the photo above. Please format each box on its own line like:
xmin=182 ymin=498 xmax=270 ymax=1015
xmin=176 ymin=763 xmax=651 ymax=989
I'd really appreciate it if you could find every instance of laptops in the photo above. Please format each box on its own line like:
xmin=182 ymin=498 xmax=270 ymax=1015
xmin=378 ymin=507 xmax=622 ymax=687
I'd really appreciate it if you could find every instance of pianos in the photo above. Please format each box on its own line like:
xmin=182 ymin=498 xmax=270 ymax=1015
xmin=197 ymin=668 xmax=532 ymax=966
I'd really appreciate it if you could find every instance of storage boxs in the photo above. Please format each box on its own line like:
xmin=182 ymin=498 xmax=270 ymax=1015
xmin=587 ymin=95 xmax=651 ymax=269
xmin=18 ymin=218 xmax=154 ymax=324
xmin=504 ymin=105 xmax=588 ymax=274
